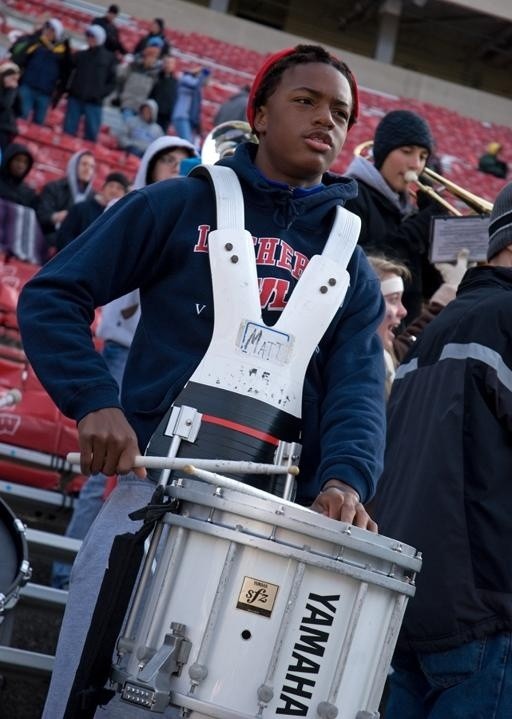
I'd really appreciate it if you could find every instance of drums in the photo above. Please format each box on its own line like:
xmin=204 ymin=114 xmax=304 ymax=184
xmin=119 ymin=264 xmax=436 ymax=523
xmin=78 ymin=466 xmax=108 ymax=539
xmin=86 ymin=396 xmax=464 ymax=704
xmin=108 ymin=477 xmax=423 ymax=719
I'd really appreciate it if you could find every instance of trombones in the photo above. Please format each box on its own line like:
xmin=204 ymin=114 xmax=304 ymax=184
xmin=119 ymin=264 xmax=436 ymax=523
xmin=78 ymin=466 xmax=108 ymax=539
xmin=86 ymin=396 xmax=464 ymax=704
xmin=354 ymin=138 xmax=494 ymax=217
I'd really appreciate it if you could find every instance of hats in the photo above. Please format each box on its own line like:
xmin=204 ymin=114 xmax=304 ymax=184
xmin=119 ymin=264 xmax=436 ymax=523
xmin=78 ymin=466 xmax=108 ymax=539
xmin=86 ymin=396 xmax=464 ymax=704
xmin=485 ymin=178 xmax=512 ymax=263
xmin=245 ymin=41 xmax=360 ymax=137
xmin=372 ymin=110 xmax=432 ymax=171
xmin=42 ymin=17 xmax=166 ymax=55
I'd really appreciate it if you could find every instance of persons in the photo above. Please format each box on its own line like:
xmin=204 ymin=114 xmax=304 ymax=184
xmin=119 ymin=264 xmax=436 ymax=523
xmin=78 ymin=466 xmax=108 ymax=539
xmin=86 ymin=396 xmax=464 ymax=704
xmin=371 ymin=180 xmax=512 ymax=719
xmin=341 ymin=107 xmax=454 ymax=337
xmin=12 ymin=39 xmax=391 ymax=719
xmin=477 ymin=142 xmax=508 ymax=179
xmin=0 ymin=3 xmax=254 ymax=261
xmin=366 ymin=248 xmax=478 ymax=403
xmin=49 ymin=134 xmax=200 ymax=591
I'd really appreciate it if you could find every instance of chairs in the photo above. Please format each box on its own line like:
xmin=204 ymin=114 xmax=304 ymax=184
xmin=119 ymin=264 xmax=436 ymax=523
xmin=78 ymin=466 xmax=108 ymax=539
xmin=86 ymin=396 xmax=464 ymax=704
xmin=0 ymin=0 xmax=512 ymax=501
